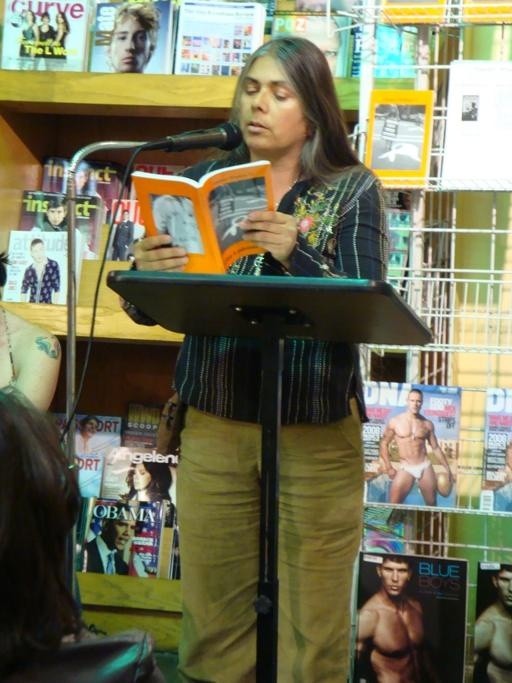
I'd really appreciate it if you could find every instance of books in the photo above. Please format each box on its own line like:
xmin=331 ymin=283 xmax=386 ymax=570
xmin=365 ymin=21 xmax=512 ymax=303
xmin=1 ymin=157 xmax=184 ymax=305
xmin=352 ymin=379 xmax=512 ymax=682
xmin=44 ymin=400 xmax=178 ymax=580
xmin=1 ymin=0 xmax=367 ymax=78
xmin=130 ymin=160 xmax=277 ymax=274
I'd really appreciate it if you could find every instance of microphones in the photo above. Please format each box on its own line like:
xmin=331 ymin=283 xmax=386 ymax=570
xmin=165 ymin=122 xmax=242 ymax=152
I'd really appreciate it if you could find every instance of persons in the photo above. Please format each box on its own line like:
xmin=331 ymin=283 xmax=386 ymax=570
xmin=119 ymin=38 xmax=388 ymax=681
xmin=0 ymin=251 xmax=62 ymax=413
xmin=0 ymin=386 xmax=165 ymax=683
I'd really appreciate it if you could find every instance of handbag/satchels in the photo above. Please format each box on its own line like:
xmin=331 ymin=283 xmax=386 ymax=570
xmin=153 ymin=392 xmax=184 ymax=456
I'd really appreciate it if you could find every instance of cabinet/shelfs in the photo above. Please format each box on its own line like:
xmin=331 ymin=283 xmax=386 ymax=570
xmin=0 ymin=0 xmax=512 ymax=683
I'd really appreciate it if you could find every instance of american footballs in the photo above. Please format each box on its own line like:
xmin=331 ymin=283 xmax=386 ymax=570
xmin=437 ymin=473 xmax=452 ymax=496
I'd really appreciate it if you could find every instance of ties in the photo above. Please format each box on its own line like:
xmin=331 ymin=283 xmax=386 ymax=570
xmin=106 ymin=554 xmax=115 ymax=574
